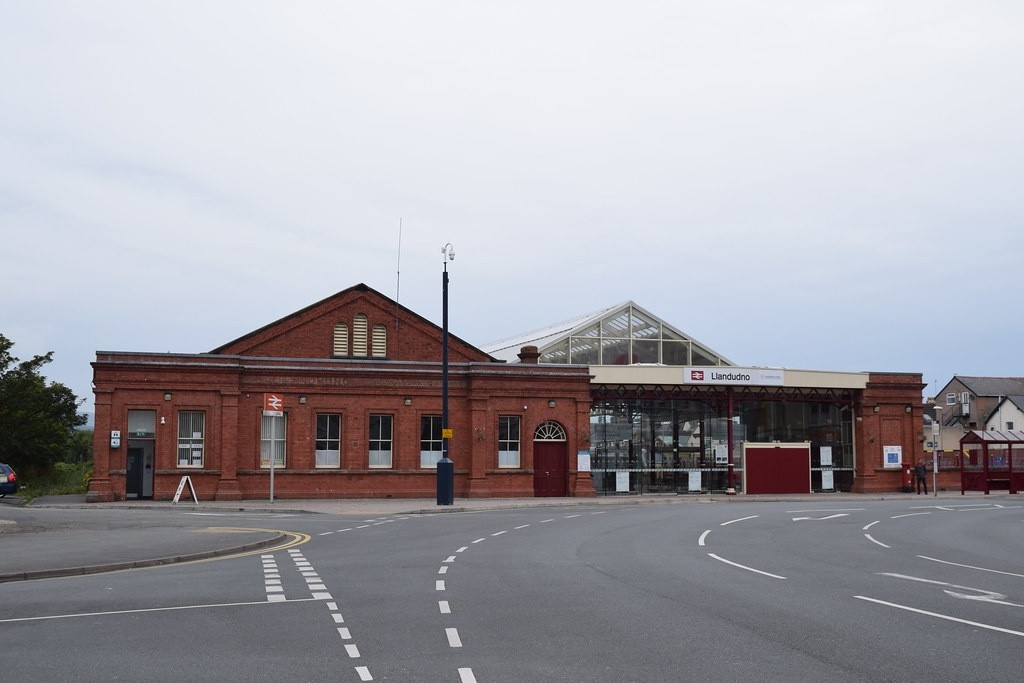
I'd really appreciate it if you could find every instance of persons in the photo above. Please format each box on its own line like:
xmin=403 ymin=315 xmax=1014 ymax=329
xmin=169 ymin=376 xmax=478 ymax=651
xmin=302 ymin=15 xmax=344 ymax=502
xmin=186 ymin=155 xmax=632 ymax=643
xmin=914 ymin=458 xmax=928 ymax=495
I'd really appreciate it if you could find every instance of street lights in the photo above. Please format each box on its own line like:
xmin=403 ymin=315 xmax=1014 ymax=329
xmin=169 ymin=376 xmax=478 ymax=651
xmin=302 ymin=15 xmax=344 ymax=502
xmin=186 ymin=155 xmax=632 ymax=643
xmin=436 ymin=243 xmax=455 ymax=506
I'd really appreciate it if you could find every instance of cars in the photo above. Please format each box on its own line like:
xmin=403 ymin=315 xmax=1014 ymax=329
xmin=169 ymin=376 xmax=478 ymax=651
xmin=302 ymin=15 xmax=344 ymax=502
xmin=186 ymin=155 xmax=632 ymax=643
xmin=0 ymin=463 xmax=19 ymax=498
